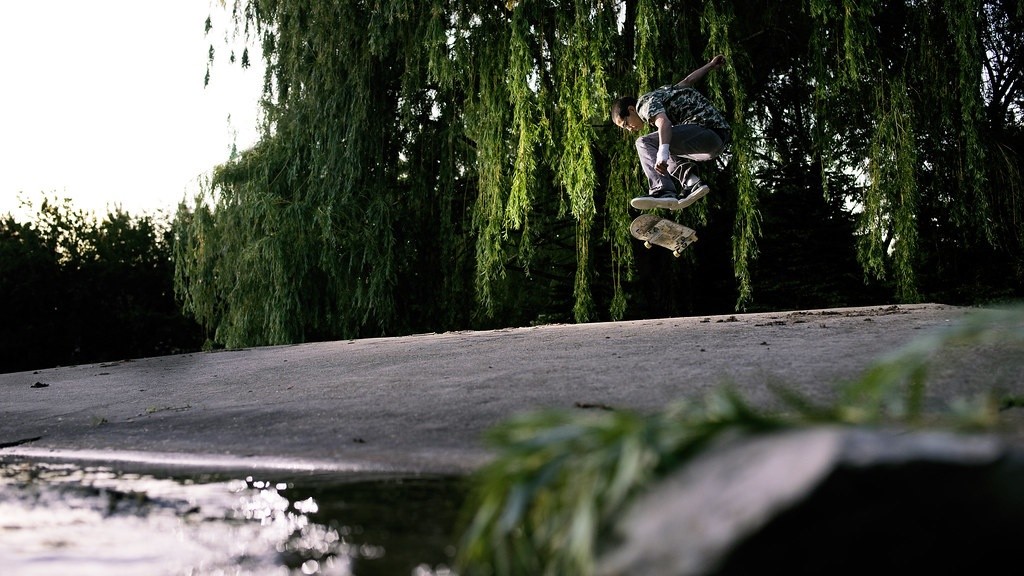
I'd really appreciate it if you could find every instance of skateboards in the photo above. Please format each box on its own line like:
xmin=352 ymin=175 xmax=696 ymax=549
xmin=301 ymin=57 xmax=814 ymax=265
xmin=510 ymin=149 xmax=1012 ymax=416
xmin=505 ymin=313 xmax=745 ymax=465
xmin=630 ymin=214 xmax=699 ymax=257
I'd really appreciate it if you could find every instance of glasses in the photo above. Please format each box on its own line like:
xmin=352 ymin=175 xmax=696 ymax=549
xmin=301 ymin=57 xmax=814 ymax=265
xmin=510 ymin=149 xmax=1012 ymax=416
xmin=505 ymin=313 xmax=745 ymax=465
xmin=622 ymin=114 xmax=628 ymax=129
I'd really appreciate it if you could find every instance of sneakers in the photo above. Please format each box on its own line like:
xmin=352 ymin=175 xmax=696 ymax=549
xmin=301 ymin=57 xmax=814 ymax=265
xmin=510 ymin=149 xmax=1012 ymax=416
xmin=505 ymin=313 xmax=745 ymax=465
xmin=630 ymin=190 xmax=679 ymax=209
xmin=669 ymin=179 xmax=710 ymax=210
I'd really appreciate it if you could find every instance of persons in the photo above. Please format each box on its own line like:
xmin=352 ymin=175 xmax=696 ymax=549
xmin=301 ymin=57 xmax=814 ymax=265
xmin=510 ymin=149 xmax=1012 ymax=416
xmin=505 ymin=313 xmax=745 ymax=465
xmin=612 ymin=55 xmax=731 ymax=210
